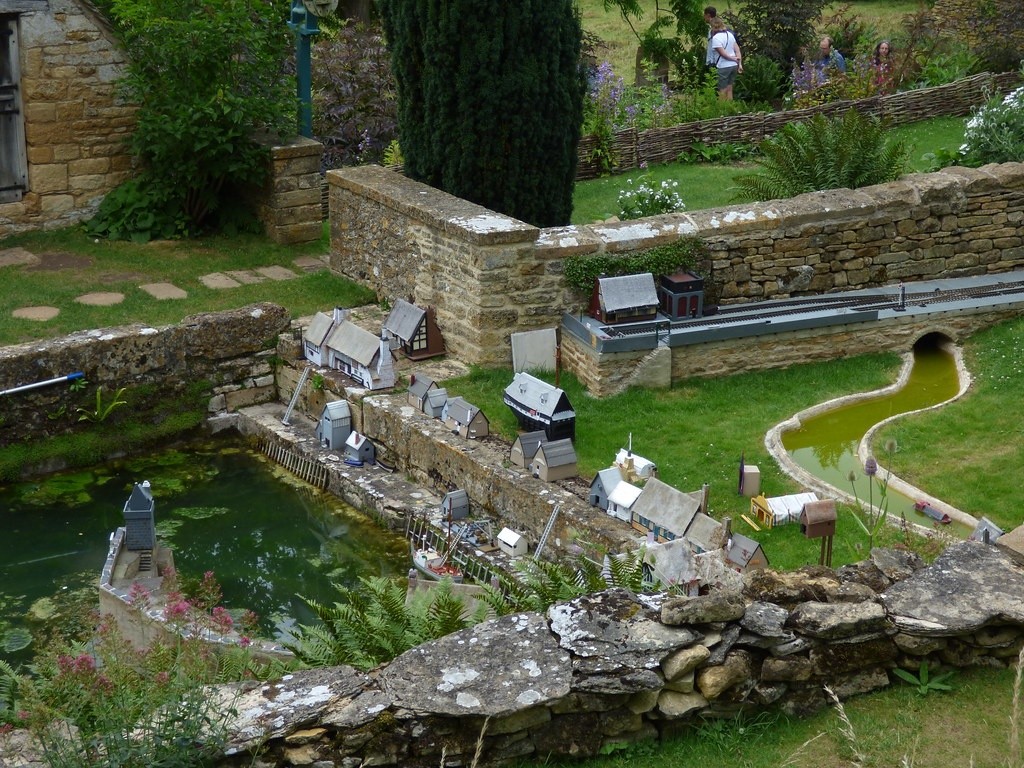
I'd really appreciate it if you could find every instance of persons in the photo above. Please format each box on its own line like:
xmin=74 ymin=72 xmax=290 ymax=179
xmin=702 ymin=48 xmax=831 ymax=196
xmin=710 ymin=17 xmax=742 ymax=102
xmin=812 ymin=36 xmax=848 ymax=73
xmin=870 ymin=40 xmax=895 ymax=96
xmin=701 ymin=5 xmax=718 ymax=72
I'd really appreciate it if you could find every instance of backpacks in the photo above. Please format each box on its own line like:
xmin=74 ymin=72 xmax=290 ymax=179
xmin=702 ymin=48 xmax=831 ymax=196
xmin=726 ymin=28 xmax=742 ymax=46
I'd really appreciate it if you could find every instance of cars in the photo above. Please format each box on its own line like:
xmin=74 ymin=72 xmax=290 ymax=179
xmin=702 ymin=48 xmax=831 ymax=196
xmin=956 ymin=83 xmax=1024 ymax=160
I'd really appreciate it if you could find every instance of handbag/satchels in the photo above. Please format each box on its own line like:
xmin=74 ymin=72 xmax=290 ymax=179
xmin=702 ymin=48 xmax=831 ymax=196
xmin=706 ymin=63 xmax=717 ymax=73
